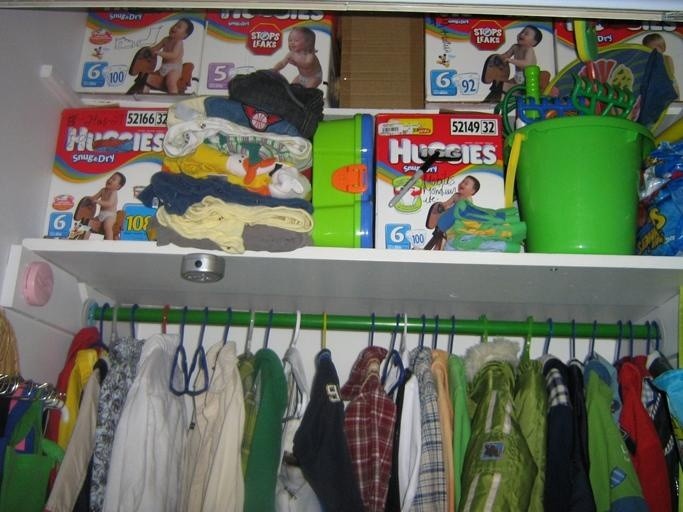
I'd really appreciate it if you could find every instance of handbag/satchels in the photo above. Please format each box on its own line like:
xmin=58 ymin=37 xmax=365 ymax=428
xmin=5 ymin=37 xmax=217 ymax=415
xmin=1 ymin=392 xmax=63 ymax=510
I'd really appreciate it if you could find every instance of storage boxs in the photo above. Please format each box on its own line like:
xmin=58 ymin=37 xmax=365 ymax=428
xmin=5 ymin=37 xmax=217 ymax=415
xmin=311 ymin=112 xmax=375 ymax=249
xmin=338 ymin=15 xmax=425 ymax=110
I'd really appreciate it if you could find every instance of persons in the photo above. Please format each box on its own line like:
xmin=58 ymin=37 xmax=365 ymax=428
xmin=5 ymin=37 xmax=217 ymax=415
xmin=500 ymin=25 xmax=543 ymax=85
xmin=441 ymin=175 xmax=480 ymax=210
xmin=86 ymin=172 xmax=126 ymax=240
xmin=147 ymin=17 xmax=194 ymax=94
xmin=641 ymin=33 xmax=680 ymax=101
xmin=269 ymin=26 xmax=324 ymax=90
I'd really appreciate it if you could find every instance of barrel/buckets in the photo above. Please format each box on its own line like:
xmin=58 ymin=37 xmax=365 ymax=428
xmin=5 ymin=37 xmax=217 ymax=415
xmin=504 ymin=116 xmax=658 ymax=255
